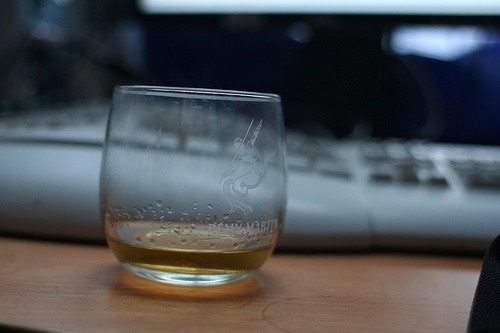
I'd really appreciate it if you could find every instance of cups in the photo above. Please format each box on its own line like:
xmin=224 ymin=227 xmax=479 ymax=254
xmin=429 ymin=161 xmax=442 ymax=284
xmin=98 ymin=86 xmax=289 ymax=287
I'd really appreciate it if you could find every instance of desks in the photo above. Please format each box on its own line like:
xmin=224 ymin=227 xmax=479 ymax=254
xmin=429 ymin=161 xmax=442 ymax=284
xmin=0 ymin=238 xmax=482 ymax=333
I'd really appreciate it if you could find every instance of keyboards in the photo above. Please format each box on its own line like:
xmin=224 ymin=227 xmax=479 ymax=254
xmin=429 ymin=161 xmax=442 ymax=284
xmin=0 ymin=100 xmax=499 ymax=252
